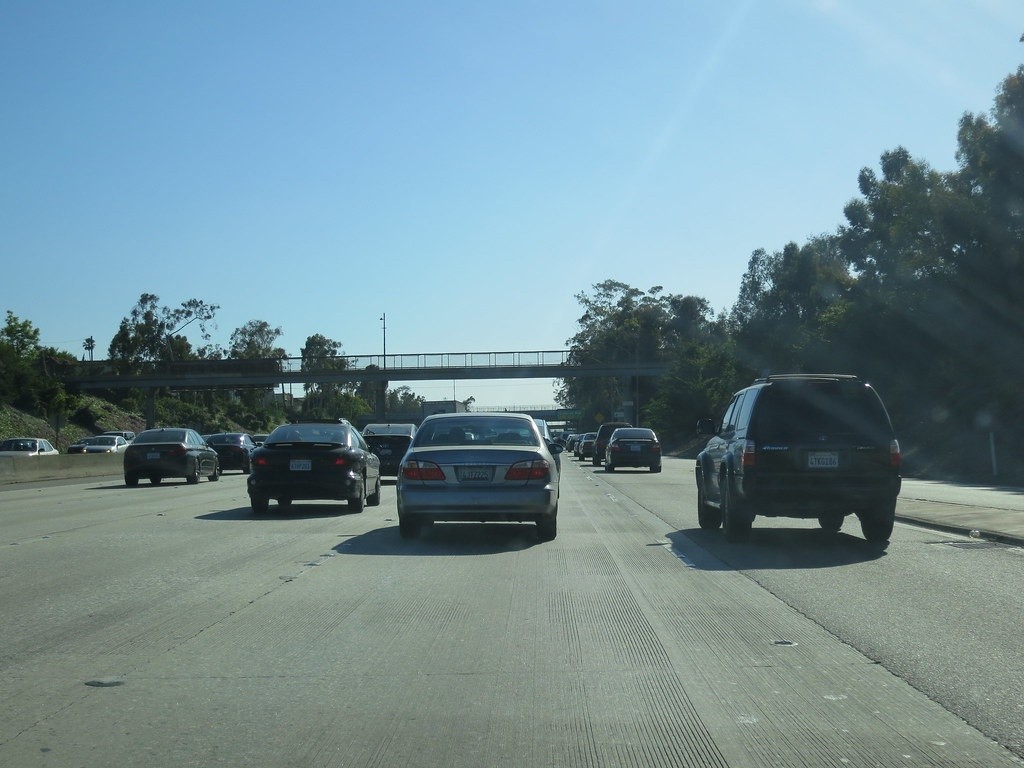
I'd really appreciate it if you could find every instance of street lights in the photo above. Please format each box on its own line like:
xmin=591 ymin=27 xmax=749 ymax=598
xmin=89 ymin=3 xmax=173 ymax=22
xmin=379 ymin=312 xmax=387 ymax=369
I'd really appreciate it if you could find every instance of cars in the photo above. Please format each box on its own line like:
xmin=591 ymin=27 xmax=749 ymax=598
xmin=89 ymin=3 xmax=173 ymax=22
xmin=592 ymin=422 xmax=634 ymax=466
xmin=81 ymin=435 xmax=129 ymax=454
xmin=206 ymin=432 xmax=262 ymax=475
xmin=605 ymin=427 xmax=662 ymax=473
xmin=578 ymin=432 xmax=598 ymax=461
xmin=0 ymin=437 xmax=59 ymax=456
xmin=68 ymin=437 xmax=93 ymax=454
xmin=123 ymin=427 xmax=221 ymax=484
xmin=360 ymin=423 xmax=418 ymax=477
xmin=103 ymin=430 xmax=136 ymax=449
xmin=552 ymin=434 xmax=586 ymax=458
xmin=251 ymin=434 xmax=271 ymax=450
xmin=518 ymin=418 xmax=562 ymax=474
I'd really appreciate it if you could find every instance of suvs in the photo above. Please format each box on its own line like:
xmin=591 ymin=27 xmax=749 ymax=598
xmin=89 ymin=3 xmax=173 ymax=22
xmin=246 ymin=418 xmax=383 ymax=512
xmin=695 ymin=373 xmax=904 ymax=542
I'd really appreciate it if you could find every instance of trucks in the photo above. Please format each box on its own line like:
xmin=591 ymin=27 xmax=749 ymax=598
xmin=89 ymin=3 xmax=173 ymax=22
xmin=395 ymin=412 xmax=564 ymax=541
xmin=421 ymin=400 xmax=466 ymax=426
